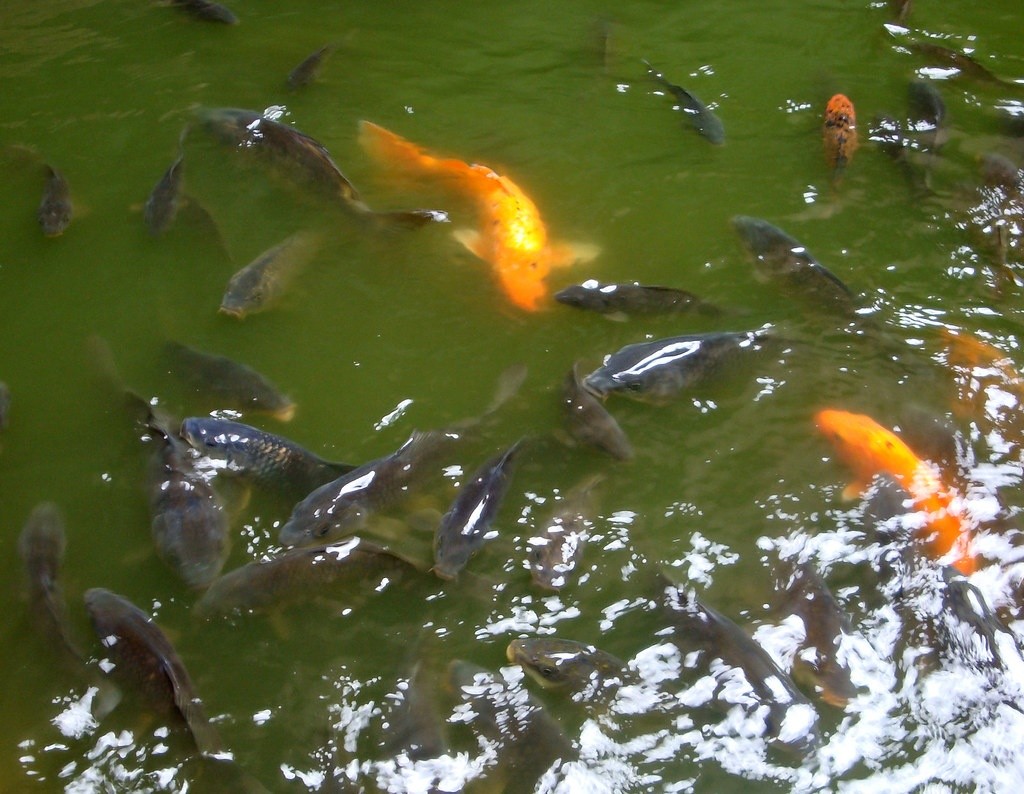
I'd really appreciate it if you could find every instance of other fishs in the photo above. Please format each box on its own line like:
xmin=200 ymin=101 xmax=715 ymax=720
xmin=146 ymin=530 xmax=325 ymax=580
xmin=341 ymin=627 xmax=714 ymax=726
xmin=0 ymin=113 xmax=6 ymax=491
xmin=14 ymin=3 xmax=1022 ymax=794
